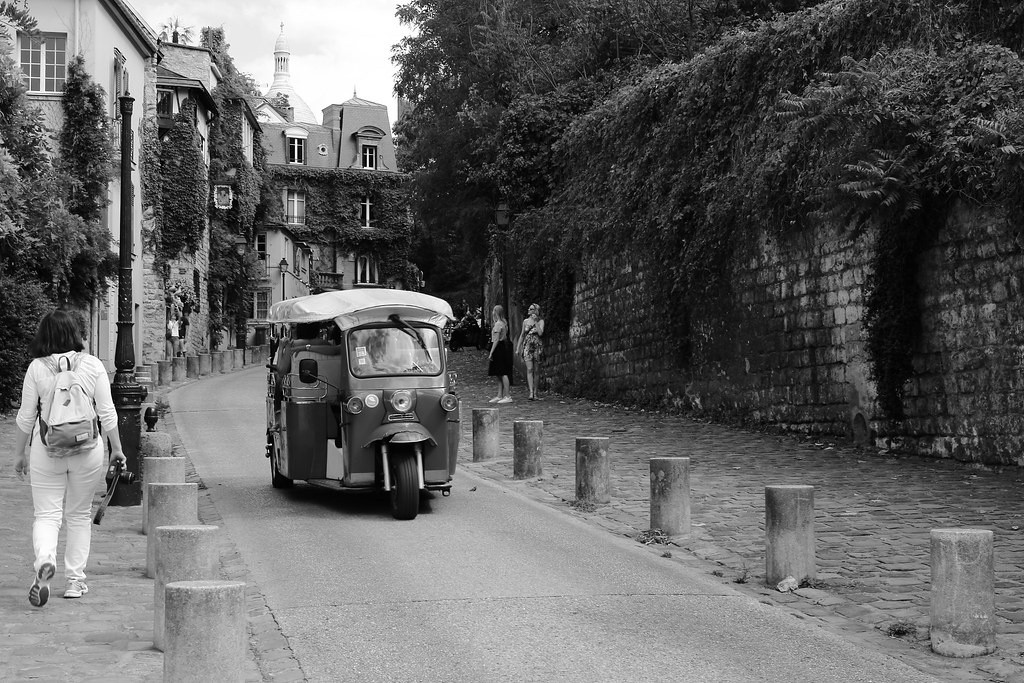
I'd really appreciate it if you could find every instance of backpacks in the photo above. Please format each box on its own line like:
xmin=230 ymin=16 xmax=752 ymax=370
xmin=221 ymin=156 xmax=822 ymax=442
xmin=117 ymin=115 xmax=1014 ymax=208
xmin=37 ymin=352 xmax=98 ymax=459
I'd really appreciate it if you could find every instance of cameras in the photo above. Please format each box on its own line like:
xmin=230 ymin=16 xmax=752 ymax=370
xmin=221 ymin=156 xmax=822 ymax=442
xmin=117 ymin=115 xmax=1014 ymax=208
xmin=105 ymin=458 xmax=136 ymax=488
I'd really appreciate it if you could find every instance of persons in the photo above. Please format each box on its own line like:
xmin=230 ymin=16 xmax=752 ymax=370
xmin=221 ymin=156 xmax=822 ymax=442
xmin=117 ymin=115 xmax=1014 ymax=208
xmin=515 ymin=304 xmax=546 ymax=400
xmin=13 ymin=310 xmax=128 ymax=607
xmin=167 ymin=312 xmax=182 ymax=357
xmin=487 ymin=305 xmax=514 ymax=404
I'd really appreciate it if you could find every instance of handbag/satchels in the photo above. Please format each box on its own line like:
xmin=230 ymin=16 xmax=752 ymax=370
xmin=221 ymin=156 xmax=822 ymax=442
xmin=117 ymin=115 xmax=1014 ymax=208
xmin=166 ymin=329 xmax=172 ymax=340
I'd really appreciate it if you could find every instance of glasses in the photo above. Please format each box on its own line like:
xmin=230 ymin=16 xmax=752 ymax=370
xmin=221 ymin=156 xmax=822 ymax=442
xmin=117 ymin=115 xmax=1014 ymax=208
xmin=528 ymin=308 xmax=534 ymax=310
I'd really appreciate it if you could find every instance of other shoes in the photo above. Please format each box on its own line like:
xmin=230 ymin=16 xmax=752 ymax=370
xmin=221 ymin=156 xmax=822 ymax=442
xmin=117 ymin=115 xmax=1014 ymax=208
xmin=528 ymin=392 xmax=534 ymax=400
xmin=534 ymin=393 xmax=538 ymax=400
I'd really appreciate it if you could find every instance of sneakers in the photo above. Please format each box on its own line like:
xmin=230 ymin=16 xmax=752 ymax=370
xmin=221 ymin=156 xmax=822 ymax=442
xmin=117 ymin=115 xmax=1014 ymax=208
xmin=64 ymin=578 xmax=88 ymax=597
xmin=489 ymin=396 xmax=502 ymax=403
xmin=28 ymin=561 xmax=55 ymax=607
xmin=497 ymin=395 xmax=513 ymax=403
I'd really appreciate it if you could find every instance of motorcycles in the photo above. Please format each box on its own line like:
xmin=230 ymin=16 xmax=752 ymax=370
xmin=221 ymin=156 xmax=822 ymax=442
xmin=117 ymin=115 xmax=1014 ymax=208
xmin=449 ymin=313 xmax=487 ymax=352
xmin=263 ymin=289 xmax=461 ymax=522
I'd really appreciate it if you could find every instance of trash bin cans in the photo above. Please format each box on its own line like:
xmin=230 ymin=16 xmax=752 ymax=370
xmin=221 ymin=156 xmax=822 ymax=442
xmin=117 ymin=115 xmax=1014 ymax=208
xmin=255 ymin=325 xmax=267 ymax=344
